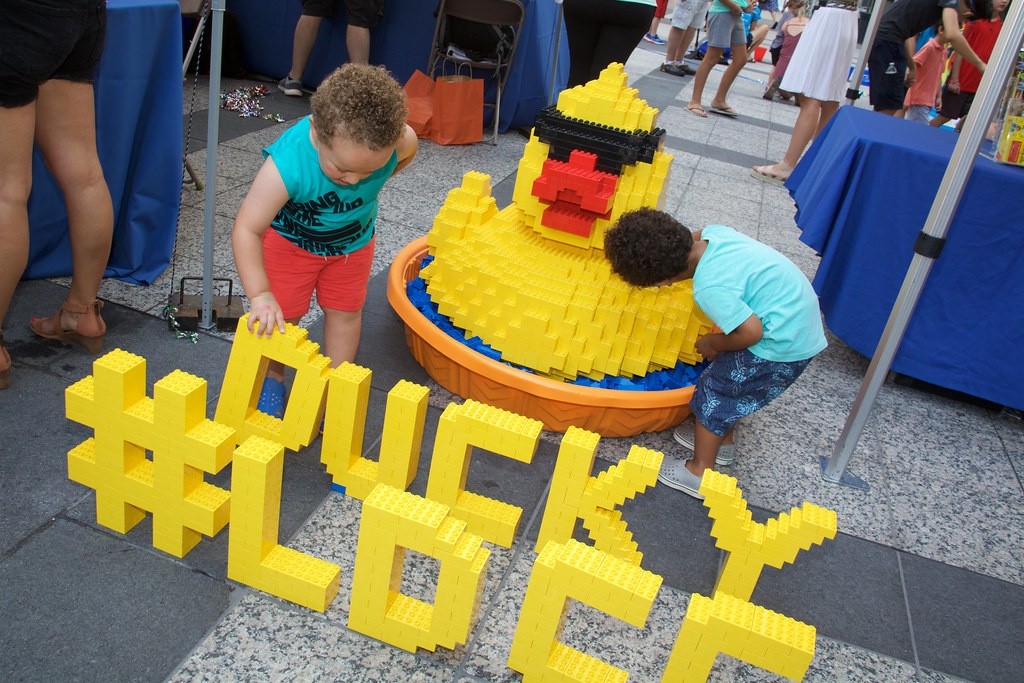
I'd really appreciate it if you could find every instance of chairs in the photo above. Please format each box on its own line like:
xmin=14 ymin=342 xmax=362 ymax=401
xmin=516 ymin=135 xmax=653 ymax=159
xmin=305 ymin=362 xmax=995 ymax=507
xmin=426 ymin=0 xmax=526 ymax=147
xmin=177 ymin=0 xmax=205 ymax=190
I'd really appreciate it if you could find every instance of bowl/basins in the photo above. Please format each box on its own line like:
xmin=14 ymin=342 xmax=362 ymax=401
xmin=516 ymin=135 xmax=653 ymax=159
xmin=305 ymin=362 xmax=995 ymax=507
xmin=387 ymin=236 xmax=697 ymax=438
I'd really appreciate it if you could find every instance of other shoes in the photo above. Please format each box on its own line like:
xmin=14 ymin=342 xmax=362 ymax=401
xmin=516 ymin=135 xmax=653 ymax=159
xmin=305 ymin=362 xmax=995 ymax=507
xmin=747 ymin=58 xmax=756 ymax=63
xmin=794 ymin=93 xmax=800 ymax=107
xmin=762 ymin=79 xmax=781 ymax=100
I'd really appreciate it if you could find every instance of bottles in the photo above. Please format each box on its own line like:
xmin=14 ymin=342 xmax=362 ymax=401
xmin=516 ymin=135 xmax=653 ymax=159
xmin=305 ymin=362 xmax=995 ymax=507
xmin=884 ymin=62 xmax=897 ymax=74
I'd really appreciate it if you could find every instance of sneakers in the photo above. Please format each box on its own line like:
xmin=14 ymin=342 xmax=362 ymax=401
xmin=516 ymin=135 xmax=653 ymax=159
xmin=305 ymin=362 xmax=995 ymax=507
xmin=660 ymin=63 xmax=696 ymax=77
xmin=319 ymin=420 xmax=325 ymax=436
xmin=674 ymin=424 xmax=737 ymax=465
xmin=258 ymin=376 xmax=287 ymax=421
xmin=278 ymin=76 xmax=303 ymax=97
xmin=643 ymin=33 xmax=667 ymax=45
xmin=658 ymin=457 xmax=706 ymax=500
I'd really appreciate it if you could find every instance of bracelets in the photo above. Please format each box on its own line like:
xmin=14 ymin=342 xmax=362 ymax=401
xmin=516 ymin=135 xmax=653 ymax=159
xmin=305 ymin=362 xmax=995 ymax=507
xmin=950 ymin=80 xmax=959 ymax=84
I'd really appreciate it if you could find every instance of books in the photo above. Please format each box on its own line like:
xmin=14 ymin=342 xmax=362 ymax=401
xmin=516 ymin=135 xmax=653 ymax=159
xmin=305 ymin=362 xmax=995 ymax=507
xmin=995 ymin=50 xmax=1024 ymax=168
xmin=447 ymin=40 xmax=497 ymax=66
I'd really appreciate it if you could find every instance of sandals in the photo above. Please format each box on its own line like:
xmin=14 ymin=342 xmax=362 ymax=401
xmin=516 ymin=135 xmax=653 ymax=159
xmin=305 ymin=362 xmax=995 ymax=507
xmin=750 ymin=160 xmax=794 ymax=187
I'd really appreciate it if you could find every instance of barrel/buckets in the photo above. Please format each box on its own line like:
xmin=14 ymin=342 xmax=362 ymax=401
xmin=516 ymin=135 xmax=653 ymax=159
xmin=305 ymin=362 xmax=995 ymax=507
xmin=753 ymin=46 xmax=767 ymax=63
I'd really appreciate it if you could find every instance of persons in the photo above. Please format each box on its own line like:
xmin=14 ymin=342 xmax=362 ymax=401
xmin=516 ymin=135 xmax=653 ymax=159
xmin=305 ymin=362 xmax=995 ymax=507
xmin=602 ymin=205 xmax=828 ymax=501
xmin=660 ymin=0 xmax=714 ymax=77
xmin=867 ymin=0 xmax=1010 ymax=127
xmin=743 ymin=0 xmax=811 ymax=107
xmin=750 ymin=0 xmax=859 ymax=193
xmin=276 ymin=0 xmax=387 ymax=95
xmin=643 ymin=0 xmax=667 ymax=45
xmin=0 ymin=0 xmax=116 ymax=391
xmin=688 ymin=0 xmax=757 ymax=117
xmin=229 ymin=62 xmax=419 ymax=439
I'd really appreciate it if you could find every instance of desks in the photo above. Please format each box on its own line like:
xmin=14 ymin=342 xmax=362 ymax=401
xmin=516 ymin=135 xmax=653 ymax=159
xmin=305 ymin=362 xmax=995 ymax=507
xmin=780 ymin=105 xmax=1024 ymax=411
xmin=221 ymin=1 xmax=573 ymax=133
xmin=20 ymin=0 xmax=183 ymax=284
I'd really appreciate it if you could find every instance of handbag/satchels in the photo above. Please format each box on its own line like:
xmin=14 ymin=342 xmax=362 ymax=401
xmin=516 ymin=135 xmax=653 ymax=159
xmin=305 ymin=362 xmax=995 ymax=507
xmin=402 ymin=55 xmax=484 ymax=146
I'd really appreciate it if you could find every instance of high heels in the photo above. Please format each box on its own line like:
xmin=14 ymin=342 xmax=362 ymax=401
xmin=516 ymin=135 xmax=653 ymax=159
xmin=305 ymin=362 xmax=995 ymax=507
xmin=0 ymin=330 xmax=12 ymax=389
xmin=30 ymin=299 xmax=107 ymax=354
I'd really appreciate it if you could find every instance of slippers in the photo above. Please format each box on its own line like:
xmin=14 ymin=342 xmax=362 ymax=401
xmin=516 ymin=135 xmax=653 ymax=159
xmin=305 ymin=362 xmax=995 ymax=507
xmin=684 ymin=107 xmax=709 ymax=118
xmin=709 ymin=106 xmax=739 ymax=116
